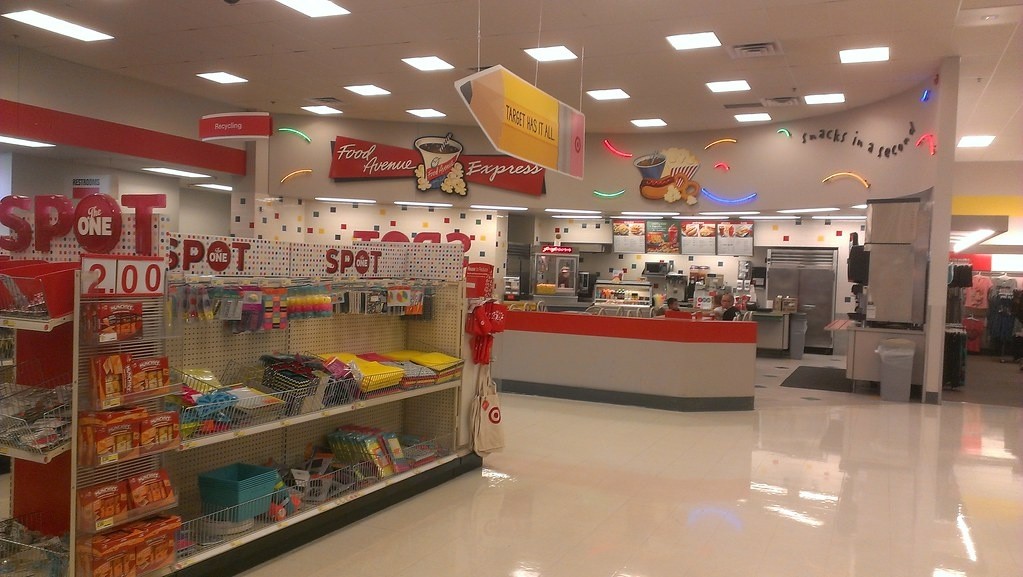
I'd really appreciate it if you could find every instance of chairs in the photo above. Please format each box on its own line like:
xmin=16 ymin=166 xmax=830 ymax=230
xmin=586 ymin=305 xmax=655 ymax=318
xmin=733 ymin=311 xmax=753 ymax=321
xmin=509 ymin=301 xmax=548 ymax=312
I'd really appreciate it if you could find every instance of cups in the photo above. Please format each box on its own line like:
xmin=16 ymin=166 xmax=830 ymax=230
xmin=668 ymin=230 xmax=678 ymax=244
xmin=719 ymin=227 xmax=725 ymax=237
xmin=729 ymin=227 xmax=734 ymax=236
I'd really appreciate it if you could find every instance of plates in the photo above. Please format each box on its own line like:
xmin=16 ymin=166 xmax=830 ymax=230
xmin=736 ymin=224 xmax=752 ymax=237
xmin=682 ymin=230 xmax=697 ymax=236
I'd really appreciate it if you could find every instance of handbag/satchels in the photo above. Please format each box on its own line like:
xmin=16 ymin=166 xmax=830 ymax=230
xmin=469 ymin=377 xmax=505 ymax=456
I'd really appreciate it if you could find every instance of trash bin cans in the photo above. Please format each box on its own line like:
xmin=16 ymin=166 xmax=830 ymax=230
xmin=878 ymin=337 xmax=917 ymax=402
xmin=789 ymin=312 xmax=807 ymax=359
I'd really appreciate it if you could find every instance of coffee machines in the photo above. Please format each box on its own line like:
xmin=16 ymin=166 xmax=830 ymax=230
xmin=846 ymin=233 xmax=926 ymax=328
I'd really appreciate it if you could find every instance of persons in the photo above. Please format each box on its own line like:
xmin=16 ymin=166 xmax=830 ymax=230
xmin=667 ymin=298 xmax=679 ymax=311
xmin=558 ymin=266 xmax=570 ymax=288
xmin=714 ymin=294 xmax=740 ymax=321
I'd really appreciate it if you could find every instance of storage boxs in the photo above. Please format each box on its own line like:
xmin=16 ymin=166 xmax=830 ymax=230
xmin=782 ymin=297 xmax=798 ymax=313
xmin=75 ymin=354 xmax=180 ymax=577
xmin=85 ymin=303 xmax=143 ymax=342
xmin=196 ymin=462 xmax=276 ymax=522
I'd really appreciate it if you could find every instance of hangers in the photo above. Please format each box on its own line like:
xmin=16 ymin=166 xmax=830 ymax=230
xmin=949 ymin=258 xmax=971 ymax=266
xmin=968 ymin=314 xmax=978 ymax=321
xmin=945 ymin=323 xmax=967 ymax=333
xmin=998 ymin=272 xmax=1010 ymax=278
xmin=999 ymin=289 xmax=1013 ymax=298
xmin=975 ymin=269 xmax=983 ymax=275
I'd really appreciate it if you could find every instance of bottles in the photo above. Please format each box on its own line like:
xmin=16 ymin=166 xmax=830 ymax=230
xmin=596 ymin=288 xmax=631 ymax=299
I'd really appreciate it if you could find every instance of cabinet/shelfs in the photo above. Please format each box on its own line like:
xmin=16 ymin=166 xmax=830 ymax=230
xmin=75 ymin=280 xmax=461 ymax=577
xmin=0 ymin=312 xmax=71 ymax=577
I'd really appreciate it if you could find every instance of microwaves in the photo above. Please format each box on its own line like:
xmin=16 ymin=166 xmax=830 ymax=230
xmin=644 ymin=259 xmax=674 ymax=275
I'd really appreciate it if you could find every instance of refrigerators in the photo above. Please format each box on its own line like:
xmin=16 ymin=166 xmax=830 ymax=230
xmin=764 ymin=248 xmax=838 ymax=348
